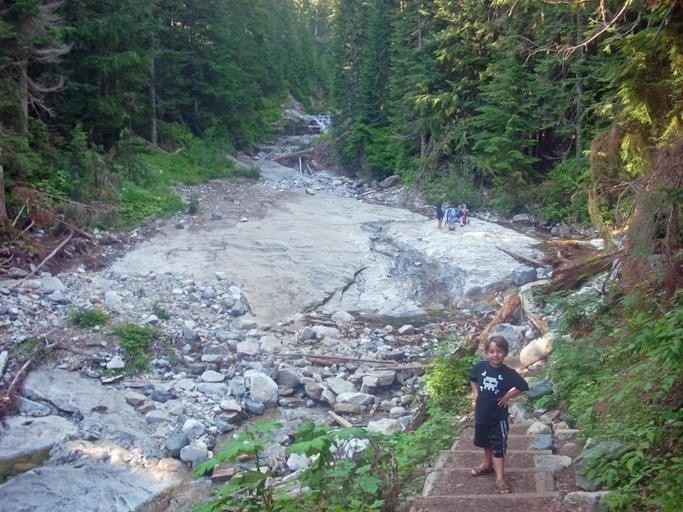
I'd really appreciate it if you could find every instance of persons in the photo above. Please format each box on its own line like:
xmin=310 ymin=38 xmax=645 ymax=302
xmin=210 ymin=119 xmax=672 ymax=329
xmin=436 ymin=201 xmax=470 ymax=231
xmin=469 ymin=336 xmax=529 ymax=493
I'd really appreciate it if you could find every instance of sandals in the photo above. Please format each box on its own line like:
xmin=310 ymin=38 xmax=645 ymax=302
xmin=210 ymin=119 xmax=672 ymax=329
xmin=472 ymin=467 xmax=494 ymax=476
xmin=495 ymin=478 xmax=511 ymax=494
